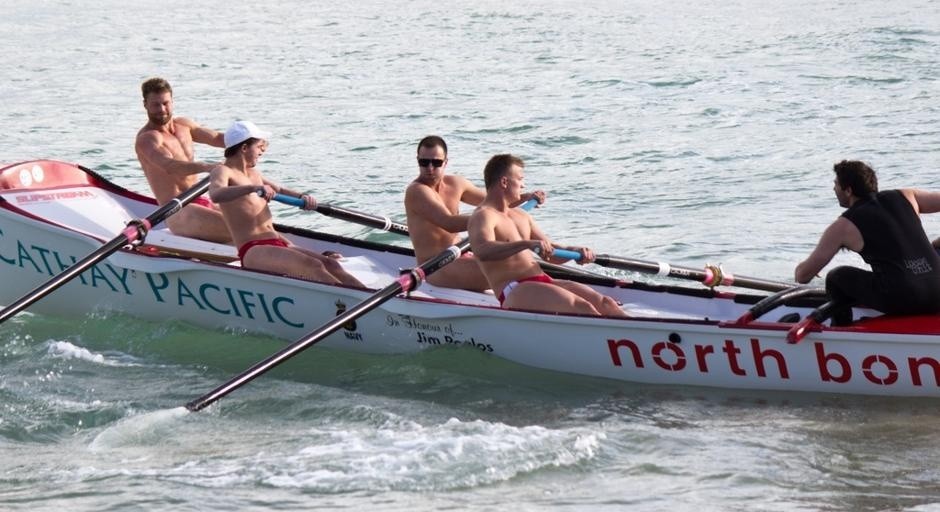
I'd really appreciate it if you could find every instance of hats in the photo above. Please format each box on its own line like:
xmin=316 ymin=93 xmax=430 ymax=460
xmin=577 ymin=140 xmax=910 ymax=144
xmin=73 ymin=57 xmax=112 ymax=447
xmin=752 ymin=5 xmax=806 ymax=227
xmin=224 ymin=121 xmax=272 ymax=152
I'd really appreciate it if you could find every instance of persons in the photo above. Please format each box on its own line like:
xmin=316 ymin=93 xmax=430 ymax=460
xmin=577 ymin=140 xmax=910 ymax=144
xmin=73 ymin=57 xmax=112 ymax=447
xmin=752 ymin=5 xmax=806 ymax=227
xmin=404 ymin=135 xmax=548 ymax=290
xmin=210 ymin=121 xmax=369 ymax=288
xmin=135 ymin=77 xmax=237 ymax=242
xmin=467 ymin=153 xmax=638 ymax=321
xmin=794 ymin=160 xmax=940 ymax=326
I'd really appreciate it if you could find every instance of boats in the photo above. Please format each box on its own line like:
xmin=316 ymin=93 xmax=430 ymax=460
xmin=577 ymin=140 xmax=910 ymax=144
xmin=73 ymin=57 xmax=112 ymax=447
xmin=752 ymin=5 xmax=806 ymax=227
xmin=1 ymin=151 xmax=940 ymax=401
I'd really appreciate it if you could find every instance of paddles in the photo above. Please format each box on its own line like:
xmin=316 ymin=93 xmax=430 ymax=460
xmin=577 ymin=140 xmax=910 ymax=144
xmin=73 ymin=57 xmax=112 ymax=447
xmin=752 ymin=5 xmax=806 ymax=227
xmin=85 ymin=191 xmax=549 ymax=450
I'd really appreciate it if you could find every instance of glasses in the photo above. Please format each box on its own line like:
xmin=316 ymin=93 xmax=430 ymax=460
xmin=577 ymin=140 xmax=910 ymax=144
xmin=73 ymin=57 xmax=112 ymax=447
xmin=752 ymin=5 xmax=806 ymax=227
xmin=418 ymin=158 xmax=443 ymax=167
